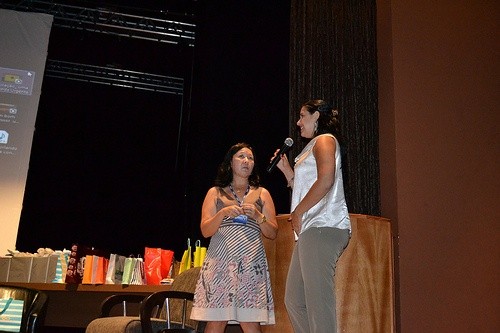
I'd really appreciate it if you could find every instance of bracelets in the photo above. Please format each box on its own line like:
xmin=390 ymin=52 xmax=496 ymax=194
xmin=287 ymin=176 xmax=295 ymax=186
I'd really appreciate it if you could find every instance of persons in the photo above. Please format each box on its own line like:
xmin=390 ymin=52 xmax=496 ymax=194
xmin=189 ymin=142 xmax=280 ymax=333
xmin=271 ymin=99 xmax=352 ymax=333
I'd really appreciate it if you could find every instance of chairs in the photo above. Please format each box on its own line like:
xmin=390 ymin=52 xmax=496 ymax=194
xmin=84 ymin=266 xmax=201 ymax=333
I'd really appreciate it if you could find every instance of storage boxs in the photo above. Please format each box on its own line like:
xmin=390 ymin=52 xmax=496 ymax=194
xmin=0 ymin=256 xmax=57 ymax=283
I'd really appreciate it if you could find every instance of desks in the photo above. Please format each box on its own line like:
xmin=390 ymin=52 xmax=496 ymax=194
xmin=0 ymin=281 xmax=172 ymax=333
xmin=260 ymin=214 xmax=395 ymax=333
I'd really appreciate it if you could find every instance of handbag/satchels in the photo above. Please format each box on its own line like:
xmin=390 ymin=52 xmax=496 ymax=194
xmin=178 ymin=237 xmax=208 ymax=275
xmin=51 ymin=252 xmax=70 ymax=283
xmin=81 ymin=254 xmax=145 ymax=285
xmin=144 ymin=247 xmax=174 ymax=284
xmin=0 ymin=297 xmax=25 ymax=333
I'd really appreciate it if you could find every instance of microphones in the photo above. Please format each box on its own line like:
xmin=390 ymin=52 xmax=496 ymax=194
xmin=266 ymin=137 xmax=294 ymax=173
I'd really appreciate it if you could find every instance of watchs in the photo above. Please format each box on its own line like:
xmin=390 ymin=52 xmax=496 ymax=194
xmin=256 ymin=215 xmax=266 ymax=225
